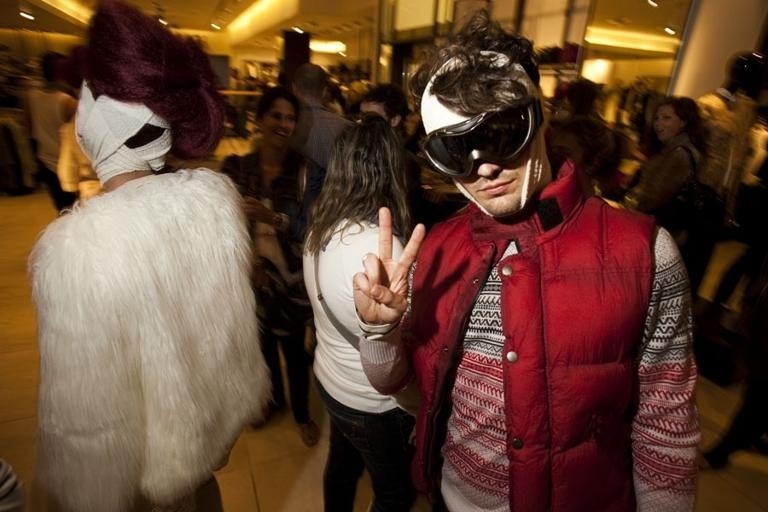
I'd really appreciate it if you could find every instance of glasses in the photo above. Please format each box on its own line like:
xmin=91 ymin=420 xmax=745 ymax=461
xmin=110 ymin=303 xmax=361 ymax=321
xmin=418 ymin=98 xmax=545 ymax=177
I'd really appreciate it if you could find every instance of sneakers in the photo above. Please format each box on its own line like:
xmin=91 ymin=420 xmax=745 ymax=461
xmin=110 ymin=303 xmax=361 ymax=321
xmin=250 ymin=403 xmax=287 ymax=428
xmin=299 ymin=420 xmax=320 ymax=446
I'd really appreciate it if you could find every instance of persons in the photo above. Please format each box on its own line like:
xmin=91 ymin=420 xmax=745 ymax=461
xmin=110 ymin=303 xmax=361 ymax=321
xmin=546 ymin=49 xmax=766 ymax=474
xmin=220 ymin=63 xmax=472 ymax=512
xmin=25 ymin=0 xmax=274 ymax=511
xmin=1 ymin=40 xmax=101 ymax=215
xmin=350 ymin=7 xmax=705 ymax=511
